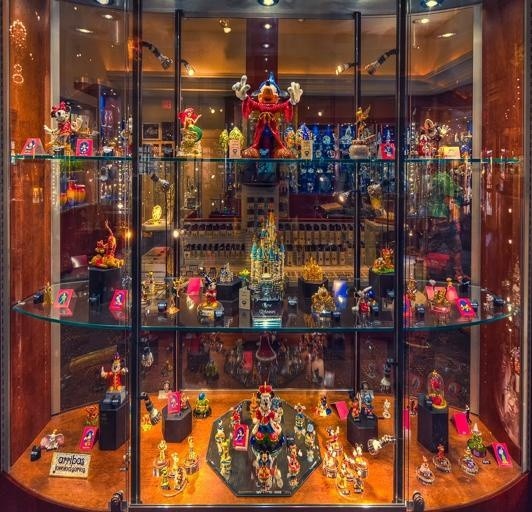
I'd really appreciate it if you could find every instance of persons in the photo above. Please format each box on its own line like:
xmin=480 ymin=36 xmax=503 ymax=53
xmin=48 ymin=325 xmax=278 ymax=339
xmin=45 ymin=73 xmax=514 ymax=490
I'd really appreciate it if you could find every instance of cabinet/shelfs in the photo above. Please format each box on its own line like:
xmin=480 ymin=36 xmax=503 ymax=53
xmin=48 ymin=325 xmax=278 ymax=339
xmin=0 ymin=0 xmax=532 ymax=512
